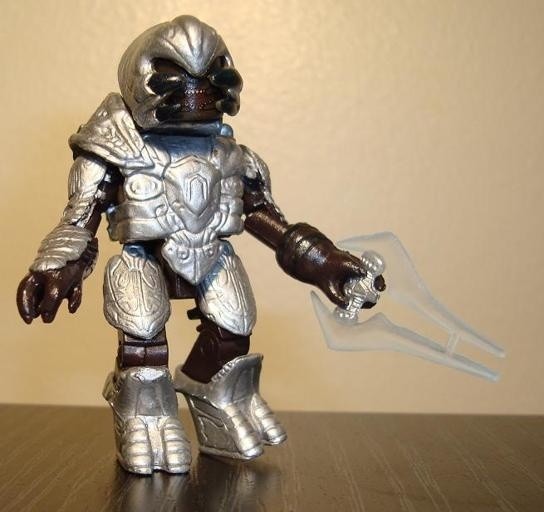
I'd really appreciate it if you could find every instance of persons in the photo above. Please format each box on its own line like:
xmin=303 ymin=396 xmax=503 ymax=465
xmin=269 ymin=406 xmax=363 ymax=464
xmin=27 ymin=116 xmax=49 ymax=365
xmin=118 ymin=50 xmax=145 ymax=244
xmin=18 ymin=13 xmax=386 ymax=477
